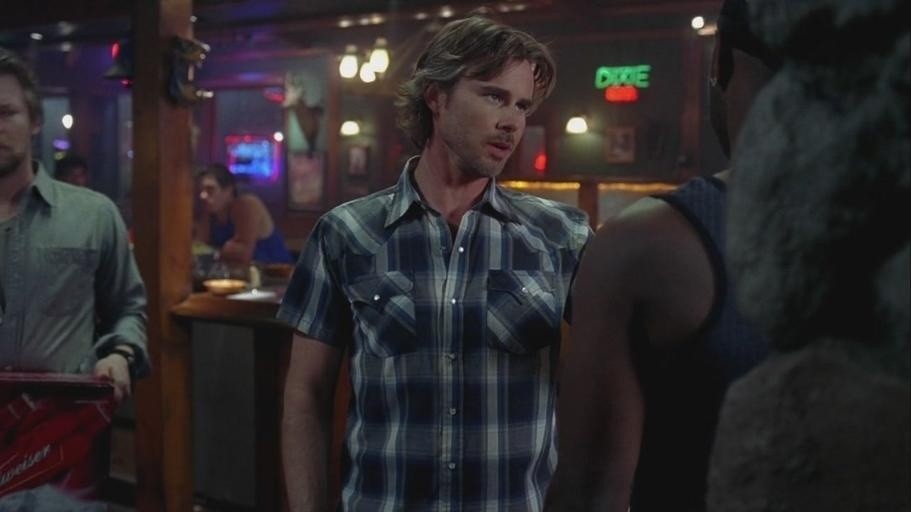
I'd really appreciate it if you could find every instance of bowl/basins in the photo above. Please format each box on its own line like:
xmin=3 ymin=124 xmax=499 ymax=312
xmin=201 ymin=276 xmax=248 ymax=296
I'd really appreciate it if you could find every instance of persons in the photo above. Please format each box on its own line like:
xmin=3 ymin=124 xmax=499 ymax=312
xmin=708 ymin=0 xmax=911 ymax=512
xmin=276 ymin=20 xmax=595 ymax=512
xmin=195 ymin=163 xmax=294 ymax=265
xmin=543 ymin=1 xmax=784 ymax=511
xmin=0 ymin=54 xmax=151 ymax=512
xmin=56 ymin=156 xmax=86 ymax=188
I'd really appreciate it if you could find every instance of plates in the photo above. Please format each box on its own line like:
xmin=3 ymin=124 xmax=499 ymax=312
xmin=0 ymin=369 xmax=118 ymax=395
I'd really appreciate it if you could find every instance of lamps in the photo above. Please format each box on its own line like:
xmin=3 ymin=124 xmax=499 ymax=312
xmin=339 ymin=43 xmax=359 ymax=80
xmin=565 ymin=114 xmax=587 ymax=135
xmin=359 ymin=57 xmax=376 ymax=82
xmin=368 ymin=37 xmax=391 ymax=73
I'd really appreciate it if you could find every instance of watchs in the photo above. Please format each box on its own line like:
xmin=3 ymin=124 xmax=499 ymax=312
xmin=113 ymin=348 xmax=137 ymax=371
